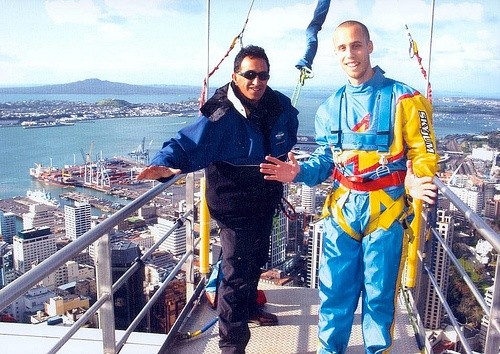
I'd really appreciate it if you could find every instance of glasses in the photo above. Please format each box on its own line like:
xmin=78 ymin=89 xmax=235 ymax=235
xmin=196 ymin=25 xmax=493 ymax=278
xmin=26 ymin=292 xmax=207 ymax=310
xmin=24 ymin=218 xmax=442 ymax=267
xmin=238 ymin=70 xmax=270 ymax=80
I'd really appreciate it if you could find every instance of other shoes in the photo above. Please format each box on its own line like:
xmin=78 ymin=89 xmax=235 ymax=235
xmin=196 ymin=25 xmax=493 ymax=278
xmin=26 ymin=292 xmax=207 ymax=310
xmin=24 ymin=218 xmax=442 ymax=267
xmin=246 ymin=310 xmax=277 ymax=325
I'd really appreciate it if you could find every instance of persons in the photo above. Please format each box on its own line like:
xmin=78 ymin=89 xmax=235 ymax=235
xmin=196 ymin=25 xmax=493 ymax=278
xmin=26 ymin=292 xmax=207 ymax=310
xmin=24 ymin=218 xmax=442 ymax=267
xmin=260 ymin=19 xmax=442 ymax=354
xmin=141 ymin=43 xmax=300 ymax=354
